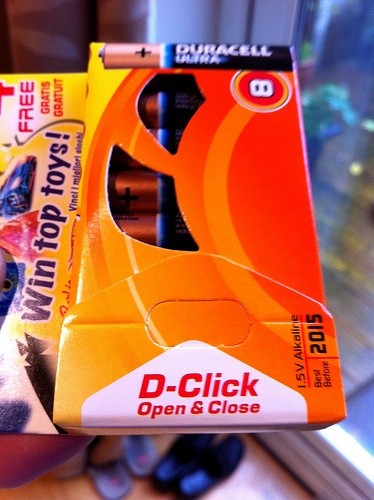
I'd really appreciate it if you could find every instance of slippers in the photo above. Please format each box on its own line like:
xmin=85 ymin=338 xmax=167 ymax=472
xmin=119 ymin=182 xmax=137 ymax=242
xmin=86 ymin=457 xmax=134 ymax=500
xmin=177 ymin=435 xmax=245 ymax=497
xmin=153 ymin=427 xmax=216 ymax=487
xmin=122 ymin=435 xmax=159 ymax=476
